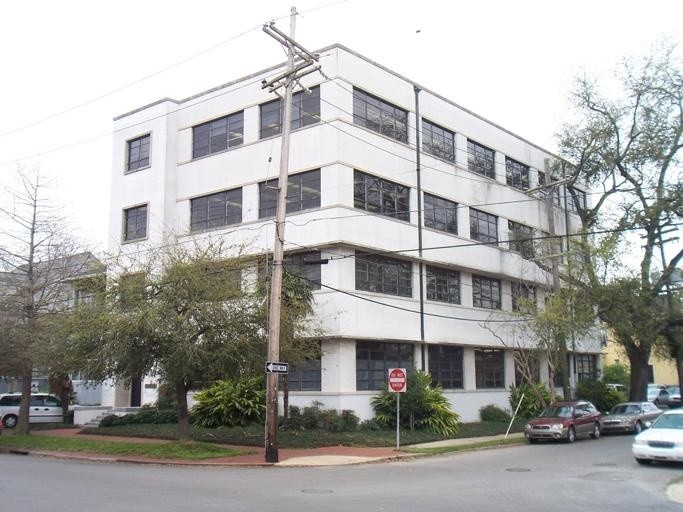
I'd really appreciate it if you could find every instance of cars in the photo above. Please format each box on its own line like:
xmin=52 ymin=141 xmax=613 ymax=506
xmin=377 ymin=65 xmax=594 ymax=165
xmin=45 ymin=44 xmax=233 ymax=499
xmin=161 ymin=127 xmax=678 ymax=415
xmin=647 ymin=383 xmax=682 ymax=407
xmin=631 ymin=408 xmax=683 ymax=464
xmin=605 ymin=382 xmax=626 ymax=392
xmin=522 ymin=399 xmax=602 ymax=443
xmin=598 ymin=402 xmax=664 ymax=434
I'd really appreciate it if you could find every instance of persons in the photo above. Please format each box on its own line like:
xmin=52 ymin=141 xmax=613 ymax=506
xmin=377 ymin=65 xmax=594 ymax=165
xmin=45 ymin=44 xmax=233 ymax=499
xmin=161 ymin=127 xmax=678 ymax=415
xmin=31 ymin=384 xmax=38 ymax=393
xmin=659 ymin=386 xmax=668 ymax=396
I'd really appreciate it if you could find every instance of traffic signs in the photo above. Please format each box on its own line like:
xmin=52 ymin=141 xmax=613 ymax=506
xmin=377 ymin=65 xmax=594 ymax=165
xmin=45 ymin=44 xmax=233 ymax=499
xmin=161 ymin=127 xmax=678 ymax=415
xmin=265 ymin=362 xmax=287 ymax=373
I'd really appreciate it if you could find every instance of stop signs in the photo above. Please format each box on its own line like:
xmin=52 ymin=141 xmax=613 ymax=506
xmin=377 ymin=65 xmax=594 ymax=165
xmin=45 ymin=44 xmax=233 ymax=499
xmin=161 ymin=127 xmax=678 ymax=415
xmin=388 ymin=368 xmax=406 ymax=391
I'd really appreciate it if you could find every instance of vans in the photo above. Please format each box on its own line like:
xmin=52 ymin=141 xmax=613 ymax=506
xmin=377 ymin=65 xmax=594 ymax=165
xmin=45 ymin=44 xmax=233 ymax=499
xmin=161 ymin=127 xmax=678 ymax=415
xmin=0 ymin=391 xmax=80 ymax=428
